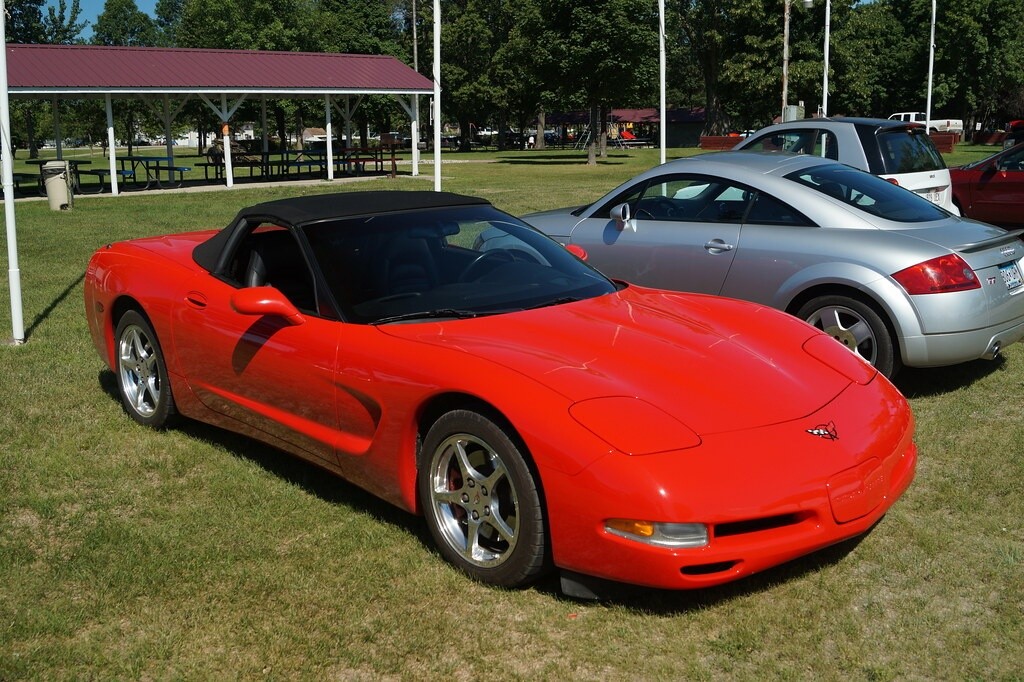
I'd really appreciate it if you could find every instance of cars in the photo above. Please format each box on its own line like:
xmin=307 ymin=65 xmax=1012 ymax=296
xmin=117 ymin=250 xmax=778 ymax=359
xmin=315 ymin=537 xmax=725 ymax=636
xmin=671 ymin=115 xmax=963 ymax=221
xmin=949 ymin=141 xmax=1023 ymax=226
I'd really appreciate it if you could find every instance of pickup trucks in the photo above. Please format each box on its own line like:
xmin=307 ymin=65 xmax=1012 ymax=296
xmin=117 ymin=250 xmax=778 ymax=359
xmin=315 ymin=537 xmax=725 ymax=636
xmin=887 ymin=111 xmax=964 ymax=136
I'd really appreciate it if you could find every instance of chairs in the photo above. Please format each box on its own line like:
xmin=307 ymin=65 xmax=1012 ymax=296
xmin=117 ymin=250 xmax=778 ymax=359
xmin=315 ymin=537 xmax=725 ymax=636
xmin=819 ymin=183 xmax=843 ymax=200
xmin=379 ymin=234 xmax=440 ymax=296
xmin=244 ymin=239 xmax=328 ymax=312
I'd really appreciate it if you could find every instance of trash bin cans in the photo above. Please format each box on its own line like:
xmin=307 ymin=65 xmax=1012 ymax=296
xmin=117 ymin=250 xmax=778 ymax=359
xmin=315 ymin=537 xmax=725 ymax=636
xmin=41 ymin=160 xmax=73 ymax=212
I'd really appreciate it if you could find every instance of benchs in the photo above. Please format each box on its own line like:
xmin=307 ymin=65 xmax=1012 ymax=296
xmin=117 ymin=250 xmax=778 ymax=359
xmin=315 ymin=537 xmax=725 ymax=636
xmin=609 ymin=138 xmax=657 ymax=149
xmin=305 ymin=160 xmax=350 ymax=181
xmin=241 ymin=160 xmax=295 ymax=180
xmin=348 ymin=156 xmax=404 ymax=179
xmin=290 ymin=161 xmax=345 ymax=181
xmin=78 ymin=169 xmax=135 ymax=195
xmin=194 ymin=163 xmax=254 ymax=185
xmin=147 ymin=166 xmax=191 ymax=188
xmin=13 ymin=172 xmax=46 ymax=198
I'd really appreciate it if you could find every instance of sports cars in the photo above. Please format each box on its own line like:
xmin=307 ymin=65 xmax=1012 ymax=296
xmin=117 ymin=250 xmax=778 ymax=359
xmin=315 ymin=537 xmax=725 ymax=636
xmin=473 ymin=146 xmax=1024 ymax=405
xmin=83 ymin=189 xmax=919 ymax=595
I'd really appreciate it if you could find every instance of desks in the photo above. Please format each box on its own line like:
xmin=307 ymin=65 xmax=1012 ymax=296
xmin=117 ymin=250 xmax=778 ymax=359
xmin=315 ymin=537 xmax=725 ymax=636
xmin=108 ymin=156 xmax=173 ymax=191
xmin=25 ymin=160 xmax=92 ymax=197
xmin=200 ymin=147 xmax=391 ymax=185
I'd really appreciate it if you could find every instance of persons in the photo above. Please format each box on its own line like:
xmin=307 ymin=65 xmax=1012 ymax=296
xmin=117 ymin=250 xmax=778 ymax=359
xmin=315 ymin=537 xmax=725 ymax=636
xmin=527 ymin=135 xmax=535 ymax=148
